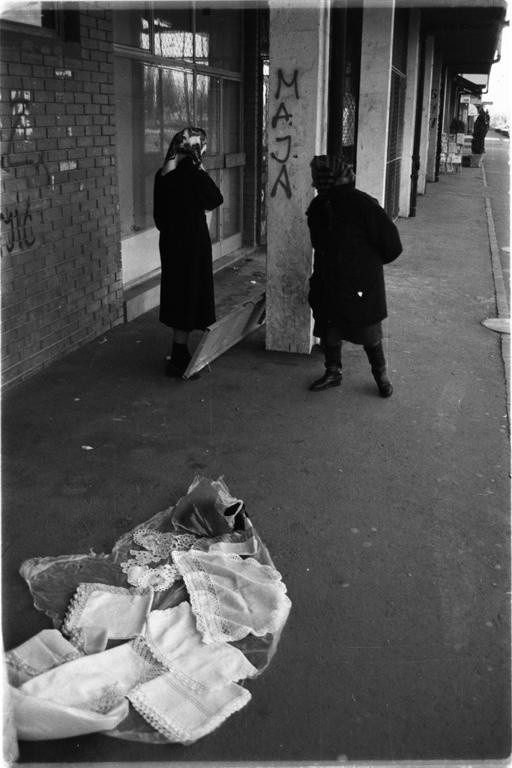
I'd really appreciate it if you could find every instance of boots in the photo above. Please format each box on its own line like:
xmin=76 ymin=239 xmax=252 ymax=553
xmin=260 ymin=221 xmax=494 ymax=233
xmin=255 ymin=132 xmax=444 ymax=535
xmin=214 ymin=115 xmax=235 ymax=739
xmin=311 ymin=362 xmax=342 ymax=390
xmin=372 ymin=366 xmax=392 ymax=397
xmin=172 ymin=342 xmax=191 ymax=365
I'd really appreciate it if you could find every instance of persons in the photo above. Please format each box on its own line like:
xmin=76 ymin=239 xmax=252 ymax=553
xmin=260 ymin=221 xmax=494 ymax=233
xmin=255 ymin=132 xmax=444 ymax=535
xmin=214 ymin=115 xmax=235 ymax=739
xmin=302 ymin=149 xmax=402 ymax=399
xmin=152 ymin=126 xmax=226 ymax=379
xmin=469 ymin=104 xmax=490 ymax=168
